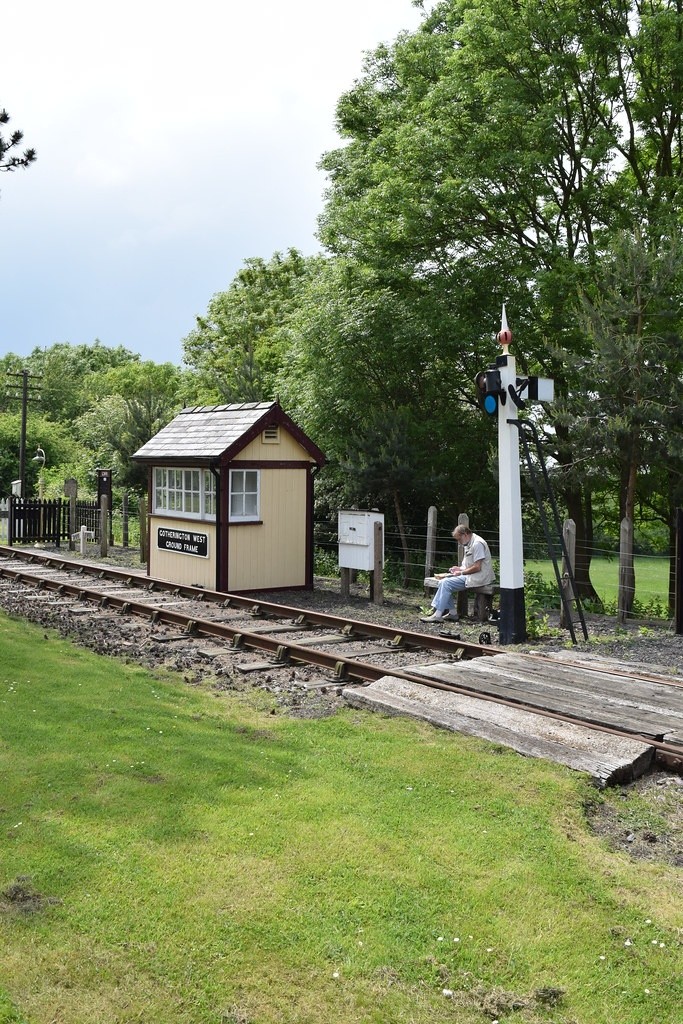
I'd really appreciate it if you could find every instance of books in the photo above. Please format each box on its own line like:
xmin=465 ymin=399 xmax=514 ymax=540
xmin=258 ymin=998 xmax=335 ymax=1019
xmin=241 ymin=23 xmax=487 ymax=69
xmin=434 ymin=573 xmax=456 ymax=580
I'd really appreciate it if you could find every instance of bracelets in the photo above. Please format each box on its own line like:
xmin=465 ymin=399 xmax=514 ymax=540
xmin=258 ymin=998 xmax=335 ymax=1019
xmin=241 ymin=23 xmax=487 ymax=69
xmin=461 ymin=571 xmax=463 ymax=575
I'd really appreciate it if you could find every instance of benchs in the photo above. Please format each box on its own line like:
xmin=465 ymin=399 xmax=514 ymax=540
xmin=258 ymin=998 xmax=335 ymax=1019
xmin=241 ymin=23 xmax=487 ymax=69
xmin=424 ymin=576 xmax=500 ymax=622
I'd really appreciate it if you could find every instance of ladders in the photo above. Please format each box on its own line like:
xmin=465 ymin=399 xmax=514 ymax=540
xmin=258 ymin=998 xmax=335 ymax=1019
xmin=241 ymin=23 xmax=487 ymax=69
xmin=505 ymin=417 xmax=589 ymax=645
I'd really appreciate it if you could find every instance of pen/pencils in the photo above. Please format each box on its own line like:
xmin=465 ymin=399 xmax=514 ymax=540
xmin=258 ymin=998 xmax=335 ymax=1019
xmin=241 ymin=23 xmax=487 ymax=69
xmin=448 ymin=568 xmax=452 ymax=573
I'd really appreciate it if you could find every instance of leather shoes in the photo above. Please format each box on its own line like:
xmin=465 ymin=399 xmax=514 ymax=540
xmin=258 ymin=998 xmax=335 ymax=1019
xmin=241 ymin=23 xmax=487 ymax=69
xmin=446 ymin=611 xmax=459 ymax=622
xmin=420 ymin=614 xmax=444 ymax=622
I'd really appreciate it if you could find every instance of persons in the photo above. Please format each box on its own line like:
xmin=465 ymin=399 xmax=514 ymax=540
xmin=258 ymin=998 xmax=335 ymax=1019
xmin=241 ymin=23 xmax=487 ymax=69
xmin=420 ymin=524 xmax=496 ymax=624
